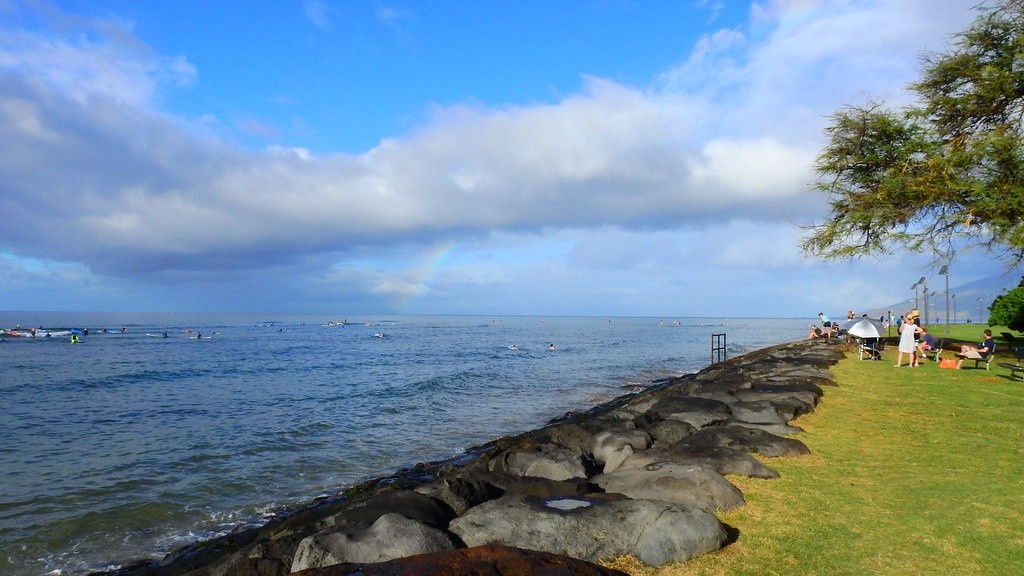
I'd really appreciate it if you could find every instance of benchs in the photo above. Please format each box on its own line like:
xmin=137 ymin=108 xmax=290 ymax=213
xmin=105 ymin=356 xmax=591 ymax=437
xmin=924 ymin=338 xmax=944 ymax=363
xmin=966 ymin=342 xmax=996 ymax=371
xmin=997 ymin=347 xmax=1024 ymax=382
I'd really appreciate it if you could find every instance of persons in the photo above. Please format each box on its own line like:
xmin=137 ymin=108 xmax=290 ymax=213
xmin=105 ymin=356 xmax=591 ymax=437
xmin=809 ymin=310 xmax=856 ymax=342
xmin=865 ymin=338 xmax=881 ymax=360
xmin=880 ymin=313 xmax=884 ymax=324
xmin=549 ymin=343 xmax=554 ymax=348
xmin=894 ymin=311 xmax=921 ymax=367
xmin=918 ymin=327 xmax=935 ymax=360
xmin=959 ymin=329 xmax=994 ymax=359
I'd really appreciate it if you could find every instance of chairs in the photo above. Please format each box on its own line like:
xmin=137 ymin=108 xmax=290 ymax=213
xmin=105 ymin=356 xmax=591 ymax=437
xmin=858 ymin=339 xmax=884 ymax=361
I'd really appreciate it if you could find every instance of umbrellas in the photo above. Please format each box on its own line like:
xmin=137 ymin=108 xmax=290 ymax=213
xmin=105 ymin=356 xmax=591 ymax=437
xmin=845 ymin=316 xmax=886 ymax=338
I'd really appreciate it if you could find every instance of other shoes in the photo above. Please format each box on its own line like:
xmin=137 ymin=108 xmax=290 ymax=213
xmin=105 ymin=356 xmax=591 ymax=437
xmin=954 ymin=352 xmax=966 ymax=360
xmin=919 ymin=357 xmax=926 ymax=361
